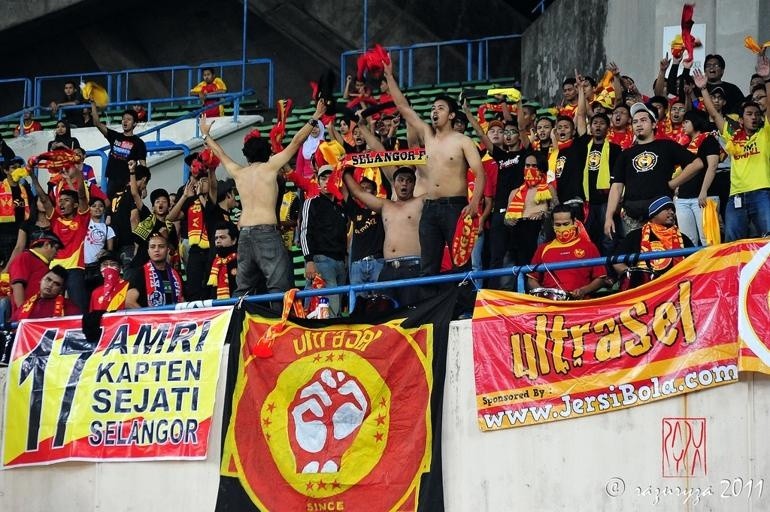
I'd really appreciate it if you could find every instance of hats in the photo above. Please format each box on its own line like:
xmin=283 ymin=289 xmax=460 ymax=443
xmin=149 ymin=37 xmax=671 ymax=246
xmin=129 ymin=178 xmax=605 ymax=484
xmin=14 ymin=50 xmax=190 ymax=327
xmin=629 ymin=102 xmax=658 ymax=122
xmin=710 ymin=85 xmax=726 ymax=98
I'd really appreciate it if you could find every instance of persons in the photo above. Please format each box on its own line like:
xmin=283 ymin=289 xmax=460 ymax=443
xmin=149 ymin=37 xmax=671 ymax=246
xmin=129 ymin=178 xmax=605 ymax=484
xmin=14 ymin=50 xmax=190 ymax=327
xmin=0 ymin=51 xmax=770 ymax=321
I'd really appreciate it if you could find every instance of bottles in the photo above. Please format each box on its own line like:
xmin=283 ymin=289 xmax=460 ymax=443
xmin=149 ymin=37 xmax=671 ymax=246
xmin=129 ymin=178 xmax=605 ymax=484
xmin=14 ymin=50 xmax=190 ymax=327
xmin=317 ymin=298 xmax=330 ymax=319
